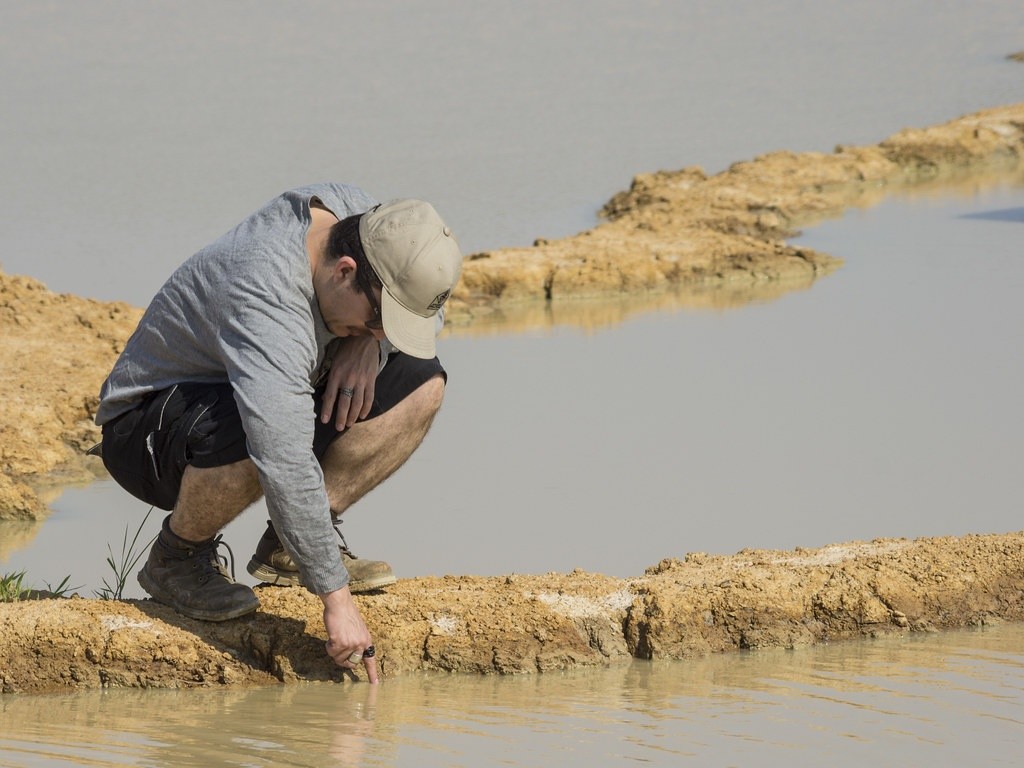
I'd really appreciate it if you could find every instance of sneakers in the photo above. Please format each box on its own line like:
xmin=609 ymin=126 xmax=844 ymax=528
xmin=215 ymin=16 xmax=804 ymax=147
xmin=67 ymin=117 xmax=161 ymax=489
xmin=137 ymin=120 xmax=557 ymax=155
xmin=137 ymin=513 xmax=261 ymax=621
xmin=247 ymin=509 xmax=397 ymax=593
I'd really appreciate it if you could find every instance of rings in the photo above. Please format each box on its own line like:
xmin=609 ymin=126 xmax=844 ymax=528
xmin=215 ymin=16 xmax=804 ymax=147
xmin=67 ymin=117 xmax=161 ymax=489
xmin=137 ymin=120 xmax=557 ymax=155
xmin=348 ymin=653 xmax=362 ymax=665
xmin=340 ymin=386 xmax=354 ymax=398
xmin=364 ymin=646 xmax=376 ymax=658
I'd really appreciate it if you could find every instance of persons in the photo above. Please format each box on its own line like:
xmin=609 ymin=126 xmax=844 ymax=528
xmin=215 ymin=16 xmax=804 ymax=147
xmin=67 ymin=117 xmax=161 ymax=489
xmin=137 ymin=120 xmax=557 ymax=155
xmin=86 ymin=181 xmax=462 ymax=686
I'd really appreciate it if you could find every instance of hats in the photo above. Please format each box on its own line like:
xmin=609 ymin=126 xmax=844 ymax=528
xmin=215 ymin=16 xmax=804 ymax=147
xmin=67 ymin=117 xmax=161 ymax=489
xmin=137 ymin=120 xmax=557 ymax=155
xmin=359 ymin=199 xmax=462 ymax=359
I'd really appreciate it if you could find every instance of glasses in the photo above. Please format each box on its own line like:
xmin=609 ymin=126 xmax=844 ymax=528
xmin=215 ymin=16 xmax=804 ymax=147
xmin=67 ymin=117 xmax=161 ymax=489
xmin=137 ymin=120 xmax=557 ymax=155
xmin=341 ymin=242 xmax=383 ymax=330
